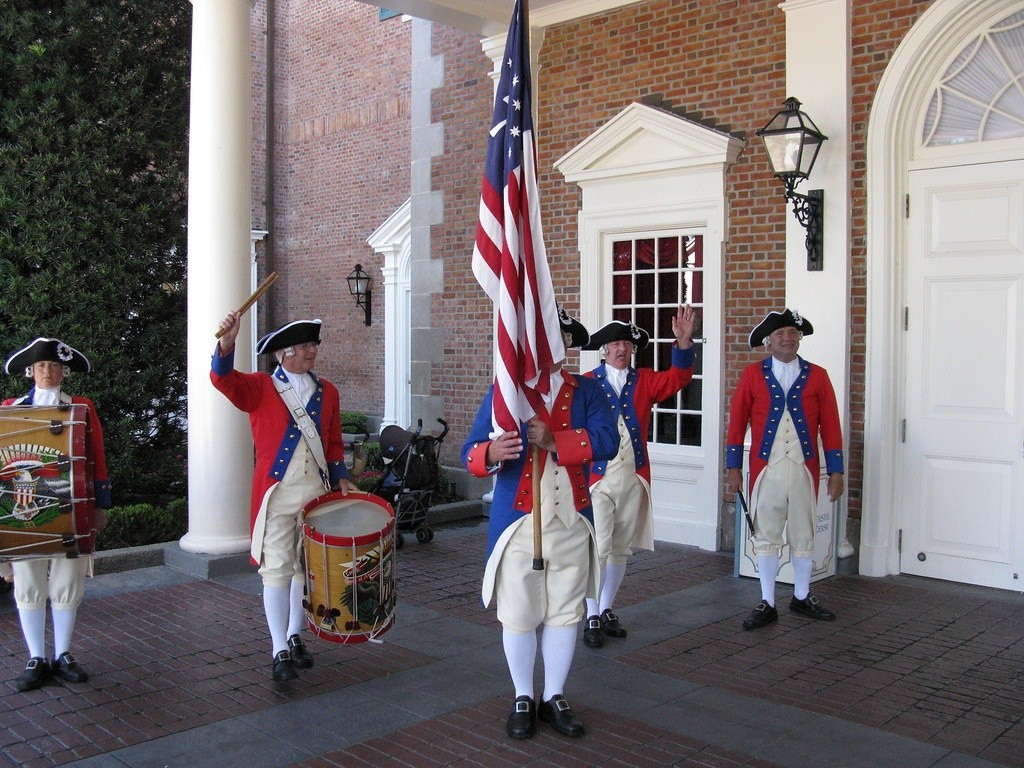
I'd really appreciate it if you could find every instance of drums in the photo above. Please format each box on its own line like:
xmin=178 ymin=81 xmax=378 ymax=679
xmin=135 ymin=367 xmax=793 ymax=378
xmin=0 ymin=402 xmax=96 ymax=565
xmin=300 ymin=490 xmax=399 ymax=646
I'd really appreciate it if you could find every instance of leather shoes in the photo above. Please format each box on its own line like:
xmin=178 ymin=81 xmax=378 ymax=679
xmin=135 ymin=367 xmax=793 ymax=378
xmin=601 ymin=608 xmax=628 ymax=638
xmin=17 ymin=657 xmax=53 ymax=690
xmin=538 ymin=693 xmax=586 ymax=737
xmin=584 ymin=615 xmax=604 ymax=647
xmin=742 ymin=599 xmax=779 ymax=629
xmin=287 ymin=633 xmax=313 ymax=670
xmin=51 ymin=651 xmax=88 ymax=683
xmin=507 ymin=695 xmax=537 ymax=739
xmin=788 ymin=588 xmax=835 ymax=620
xmin=272 ymin=650 xmax=299 ymax=682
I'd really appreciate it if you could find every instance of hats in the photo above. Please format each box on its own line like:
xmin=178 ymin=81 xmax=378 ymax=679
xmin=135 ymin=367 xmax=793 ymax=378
xmin=580 ymin=321 xmax=650 ymax=350
xmin=748 ymin=308 xmax=814 ymax=351
xmin=5 ymin=337 xmax=91 ymax=375
xmin=555 ymin=298 xmax=590 ymax=349
xmin=255 ymin=319 xmax=323 ymax=355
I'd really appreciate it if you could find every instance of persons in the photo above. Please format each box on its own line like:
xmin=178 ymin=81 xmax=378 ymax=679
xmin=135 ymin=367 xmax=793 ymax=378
xmin=581 ymin=304 xmax=698 ymax=647
xmin=210 ymin=311 xmax=360 ymax=682
xmin=726 ymin=309 xmax=844 ymax=628
xmin=461 ymin=309 xmax=620 ymax=737
xmin=0 ymin=338 xmax=111 ymax=689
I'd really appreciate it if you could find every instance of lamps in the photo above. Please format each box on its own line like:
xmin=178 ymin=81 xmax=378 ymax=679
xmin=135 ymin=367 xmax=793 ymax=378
xmin=346 ymin=262 xmax=373 ymax=326
xmin=755 ymin=96 xmax=825 ymax=273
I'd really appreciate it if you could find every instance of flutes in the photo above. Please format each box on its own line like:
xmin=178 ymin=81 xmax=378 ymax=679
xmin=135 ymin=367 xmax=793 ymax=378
xmin=737 ymin=490 xmax=756 ymax=535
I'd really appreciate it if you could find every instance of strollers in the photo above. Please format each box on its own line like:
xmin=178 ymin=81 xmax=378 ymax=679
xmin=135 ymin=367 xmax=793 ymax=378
xmin=363 ymin=416 xmax=450 ymax=551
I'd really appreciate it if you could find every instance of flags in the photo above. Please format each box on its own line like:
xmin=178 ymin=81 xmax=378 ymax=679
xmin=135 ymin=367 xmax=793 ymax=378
xmin=470 ymin=1 xmax=564 ymax=440
